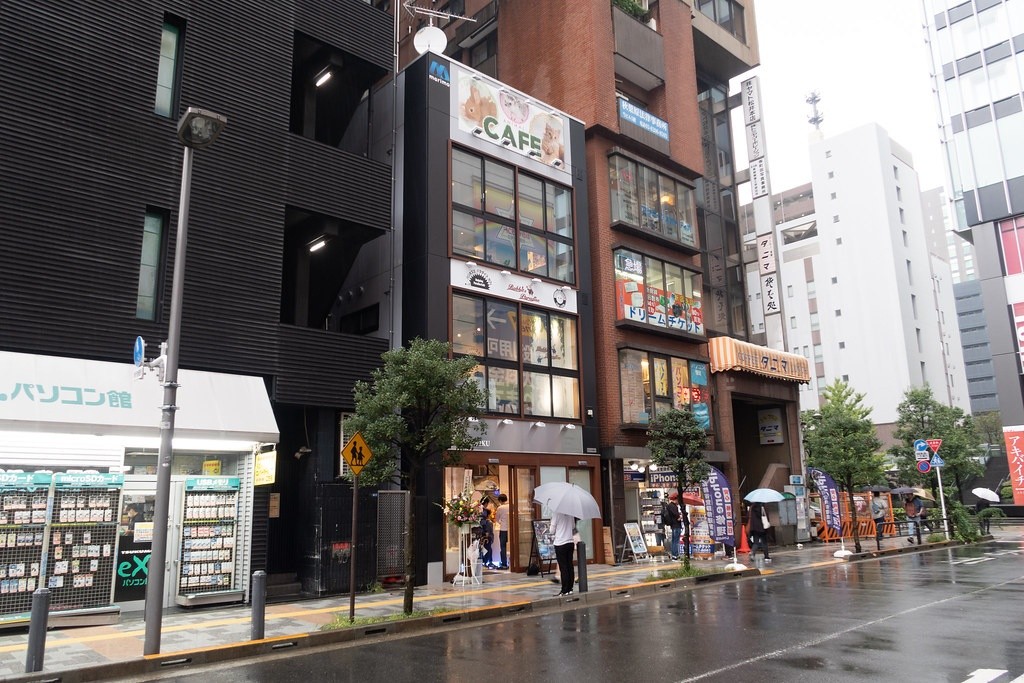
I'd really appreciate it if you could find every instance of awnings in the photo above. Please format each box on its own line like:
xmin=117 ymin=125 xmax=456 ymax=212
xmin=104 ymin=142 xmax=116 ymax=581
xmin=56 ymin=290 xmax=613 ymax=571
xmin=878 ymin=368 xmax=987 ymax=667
xmin=708 ymin=336 xmax=810 ymax=384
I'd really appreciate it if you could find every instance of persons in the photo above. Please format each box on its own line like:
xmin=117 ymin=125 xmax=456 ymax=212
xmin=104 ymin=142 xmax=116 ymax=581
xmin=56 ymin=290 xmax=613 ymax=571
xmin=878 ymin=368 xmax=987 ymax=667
xmin=479 ymin=497 xmax=498 ymax=569
xmin=653 ymin=514 xmax=666 ymax=545
xmin=667 ymin=500 xmax=684 ymax=562
xmin=977 ymin=499 xmax=991 ymax=534
xmin=550 ymin=512 xmax=580 ymax=596
xmin=496 ymin=494 xmax=509 ymax=568
xmin=903 ymin=494 xmax=924 ymax=543
xmin=915 ymin=496 xmax=933 ymax=533
xmin=749 ymin=502 xmax=772 ymax=561
xmin=872 ymin=492 xmax=888 ymax=540
xmin=123 ymin=503 xmax=145 ymax=536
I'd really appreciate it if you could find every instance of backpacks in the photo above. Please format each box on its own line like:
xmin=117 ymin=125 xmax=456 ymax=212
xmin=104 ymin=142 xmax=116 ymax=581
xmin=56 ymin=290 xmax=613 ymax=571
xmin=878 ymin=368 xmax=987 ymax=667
xmin=661 ymin=507 xmax=672 ymax=526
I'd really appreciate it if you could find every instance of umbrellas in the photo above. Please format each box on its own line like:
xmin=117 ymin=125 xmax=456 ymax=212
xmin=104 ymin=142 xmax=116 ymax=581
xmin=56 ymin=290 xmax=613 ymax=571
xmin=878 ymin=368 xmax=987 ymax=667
xmin=891 ymin=488 xmax=919 ymax=494
xmin=913 ymin=488 xmax=936 ymax=501
xmin=670 ymin=492 xmax=704 ymax=506
xmin=972 ymin=487 xmax=1000 ymax=502
xmin=744 ymin=488 xmax=785 ymax=502
xmin=534 ymin=482 xmax=601 ymax=520
xmin=863 ymin=486 xmax=892 ymax=492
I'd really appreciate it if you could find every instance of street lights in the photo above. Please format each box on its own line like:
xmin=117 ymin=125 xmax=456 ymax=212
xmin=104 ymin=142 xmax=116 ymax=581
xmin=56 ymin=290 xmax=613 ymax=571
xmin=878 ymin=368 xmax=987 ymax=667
xmin=142 ymin=105 xmax=228 ymax=656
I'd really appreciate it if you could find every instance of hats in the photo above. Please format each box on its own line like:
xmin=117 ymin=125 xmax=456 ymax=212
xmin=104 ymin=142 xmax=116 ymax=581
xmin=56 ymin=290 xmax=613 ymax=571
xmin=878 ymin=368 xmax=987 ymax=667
xmin=669 ymin=493 xmax=678 ymax=500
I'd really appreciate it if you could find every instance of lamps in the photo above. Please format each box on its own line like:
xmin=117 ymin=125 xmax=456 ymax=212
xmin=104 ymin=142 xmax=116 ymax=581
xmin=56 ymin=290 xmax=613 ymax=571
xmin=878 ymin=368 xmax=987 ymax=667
xmin=527 ymin=149 xmax=538 ymax=157
xmin=561 ymin=285 xmax=571 ymax=292
xmin=535 ymin=421 xmax=545 ymax=428
xmin=552 ymin=158 xmax=562 ymax=167
xmin=305 ymin=233 xmax=335 ymax=258
xmin=311 ymin=62 xmax=338 ymax=91
xmin=503 ymin=419 xmax=514 ymax=425
xmin=466 ymin=261 xmax=477 ymax=268
xmin=500 ymin=136 xmax=512 ymax=146
xmin=472 ymin=126 xmax=483 ymax=135
xmin=628 ymin=461 xmax=638 ymax=470
xmin=560 ymin=423 xmax=576 ymax=431
xmin=468 ymin=417 xmax=479 ymax=423
xmin=501 ymin=269 xmax=512 ymax=276
xmin=532 ymin=277 xmax=542 ymax=283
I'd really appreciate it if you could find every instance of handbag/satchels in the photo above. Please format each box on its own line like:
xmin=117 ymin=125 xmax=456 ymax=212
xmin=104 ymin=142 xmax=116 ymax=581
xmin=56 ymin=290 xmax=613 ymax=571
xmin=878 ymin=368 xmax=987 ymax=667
xmin=573 ymin=533 xmax=581 ymax=549
xmin=761 ymin=506 xmax=770 ymax=528
xmin=872 ymin=503 xmax=880 ymax=514
xmin=760 ymin=526 xmax=776 ymax=544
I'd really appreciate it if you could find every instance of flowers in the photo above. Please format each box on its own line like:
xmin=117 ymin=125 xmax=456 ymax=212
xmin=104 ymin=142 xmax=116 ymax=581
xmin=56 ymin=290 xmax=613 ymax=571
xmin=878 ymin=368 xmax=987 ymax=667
xmin=431 ymin=488 xmax=489 ymax=529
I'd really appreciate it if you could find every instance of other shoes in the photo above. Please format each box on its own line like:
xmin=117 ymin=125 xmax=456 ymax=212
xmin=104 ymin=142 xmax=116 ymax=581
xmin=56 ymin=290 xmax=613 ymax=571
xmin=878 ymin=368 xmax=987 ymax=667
xmin=496 ymin=566 xmax=507 ymax=570
xmin=553 ymin=590 xmax=574 ymax=597
xmin=750 ymin=556 xmax=754 ymax=562
xmin=672 ymin=556 xmax=684 ymax=563
xmin=488 ymin=564 xmax=498 ymax=569
xmin=907 ymin=537 xmax=914 ymax=544
xmin=764 ymin=557 xmax=772 ymax=560
